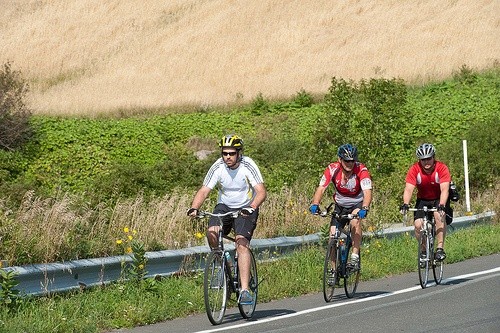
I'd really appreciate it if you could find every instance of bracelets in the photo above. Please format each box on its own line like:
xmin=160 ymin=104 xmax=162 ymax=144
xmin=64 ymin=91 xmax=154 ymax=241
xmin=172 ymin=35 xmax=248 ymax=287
xmin=250 ymin=204 xmax=257 ymax=208
xmin=362 ymin=206 xmax=367 ymax=209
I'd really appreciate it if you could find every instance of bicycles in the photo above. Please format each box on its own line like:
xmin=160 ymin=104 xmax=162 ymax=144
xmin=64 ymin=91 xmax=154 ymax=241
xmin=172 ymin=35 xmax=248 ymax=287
xmin=400 ymin=207 xmax=449 ymax=290
xmin=308 ymin=207 xmax=367 ymax=303
xmin=189 ymin=209 xmax=264 ymax=325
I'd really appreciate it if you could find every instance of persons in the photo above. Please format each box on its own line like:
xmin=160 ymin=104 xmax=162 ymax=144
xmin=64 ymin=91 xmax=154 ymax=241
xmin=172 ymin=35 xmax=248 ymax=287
xmin=310 ymin=144 xmax=372 ymax=285
xmin=399 ymin=144 xmax=451 ymax=267
xmin=186 ymin=135 xmax=266 ymax=304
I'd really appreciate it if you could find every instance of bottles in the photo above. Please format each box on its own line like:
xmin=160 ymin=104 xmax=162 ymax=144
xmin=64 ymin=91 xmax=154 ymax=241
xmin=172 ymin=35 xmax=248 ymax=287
xmin=340 ymin=238 xmax=346 ymax=261
xmin=451 ymin=181 xmax=457 ymax=199
xmin=225 ymin=252 xmax=233 ymax=268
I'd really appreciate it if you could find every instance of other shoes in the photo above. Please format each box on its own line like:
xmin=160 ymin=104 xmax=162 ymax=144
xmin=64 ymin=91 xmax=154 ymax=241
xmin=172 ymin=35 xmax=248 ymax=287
xmin=435 ymin=248 xmax=445 ymax=260
xmin=327 ymin=272 xmax=339 ymax=287
xmin=419 ymin=250 xmax=428 ymax=269
xmin=348 ymin=254 xmax=360 ymax=271
xmin=240 ymin=290 xmax=253 ymax=305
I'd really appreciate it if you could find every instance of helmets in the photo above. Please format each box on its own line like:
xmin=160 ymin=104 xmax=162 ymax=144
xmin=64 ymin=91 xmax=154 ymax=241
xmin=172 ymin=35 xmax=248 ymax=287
xmin=416 ymin=143 xmax=437 ymax=159
xmin=337 ymin=143 xmax=358 ymax=161
xmin=219 ymin=135 xmax=244 ymax=148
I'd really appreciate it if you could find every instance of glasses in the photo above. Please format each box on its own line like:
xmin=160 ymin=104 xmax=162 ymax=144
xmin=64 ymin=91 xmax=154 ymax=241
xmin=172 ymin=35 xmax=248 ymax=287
xmin=222 ymin=151 xmax=237 ymax=156
xmin=343 ymin=159 xmax=355 ymax=163
xmin=421 ymin=157 xmax=432 ymax=161
